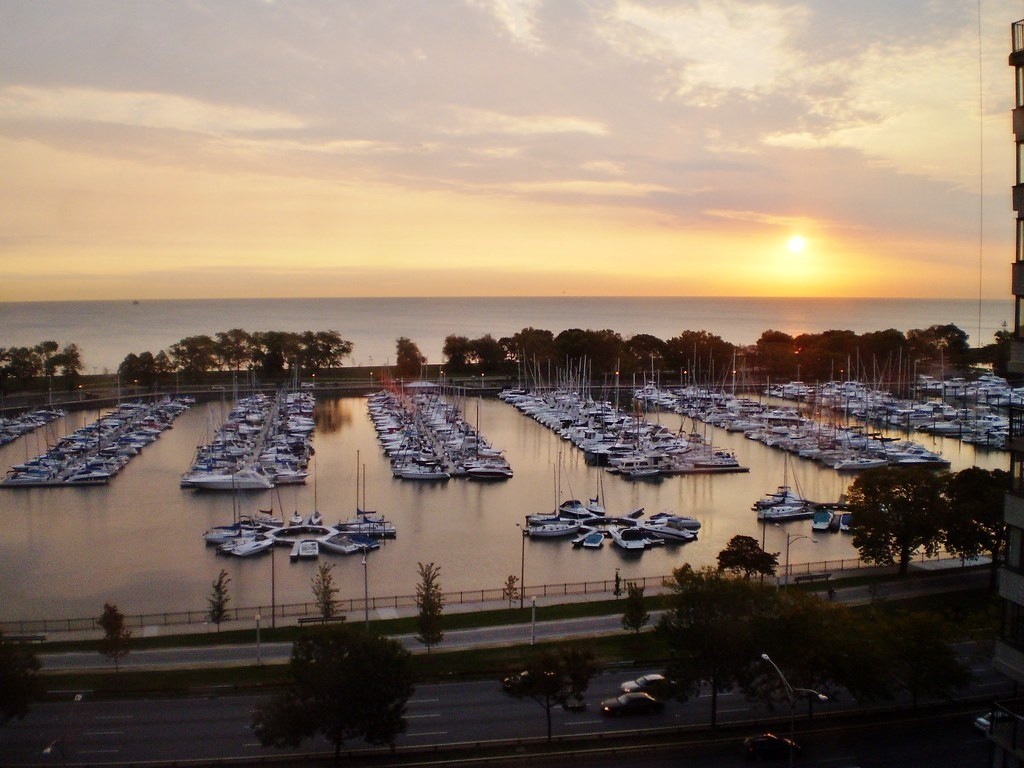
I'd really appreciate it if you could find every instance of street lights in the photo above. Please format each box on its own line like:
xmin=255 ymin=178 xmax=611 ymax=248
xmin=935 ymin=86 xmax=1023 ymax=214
xmin=761 ymin=654 xmax=828 ymax=768
xmin=515 ymin=523 xmax=525 ymax=606
xmin=532 ymin=596 xmax=535 ymax=645
xmin=361 ymin=546 xmax=368 ymax=622
xmin=761 ymin=512 xmax=780 ymax=587
xmin=43 ymin=694 xmax=83 ymax=768
xmin=256 ymin=616 xmax=260 ymax=662
xmin=774 ymin=522 xmax=819 ymax=595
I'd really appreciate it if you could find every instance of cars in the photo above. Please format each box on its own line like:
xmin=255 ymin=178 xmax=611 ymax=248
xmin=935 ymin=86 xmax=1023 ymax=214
xmin=600 ymin=692 xmax=665 ymax=718
xmin=498 ymin=670 xmax=562 ymax=693
xmin=975 ymin=712 xmax=1009 ymax=732
xmin=620 ymin=672 xmax=670 ymax=696
xmin=737 ymin=731 xmax=802 ymax=762
xmin=784 ymin=688 xmax=829 ymax=713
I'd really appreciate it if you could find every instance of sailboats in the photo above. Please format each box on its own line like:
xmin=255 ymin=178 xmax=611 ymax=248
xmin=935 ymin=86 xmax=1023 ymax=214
xmin=1 ymin=332 xmax=1024 ymax=559
